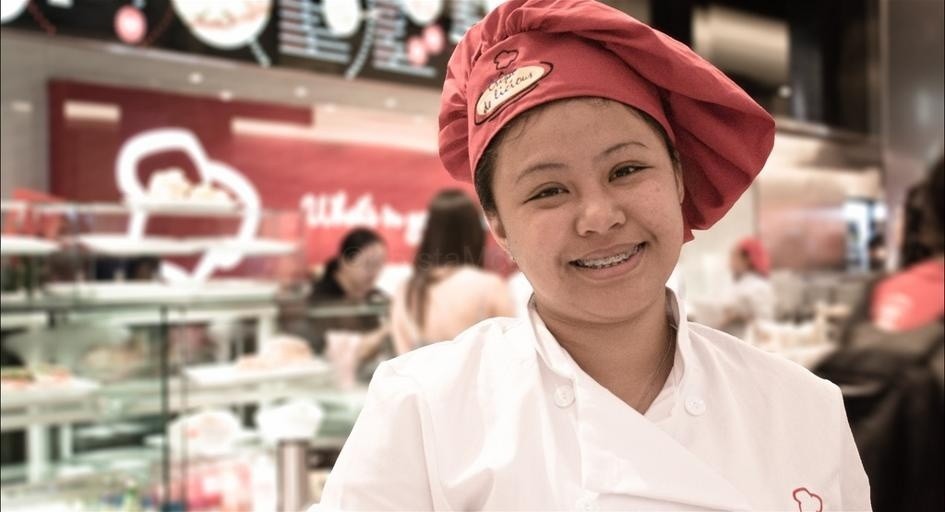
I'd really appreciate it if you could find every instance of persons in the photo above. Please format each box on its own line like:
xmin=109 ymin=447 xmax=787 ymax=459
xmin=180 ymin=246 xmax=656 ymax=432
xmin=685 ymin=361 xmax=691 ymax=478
xmin=867 ymin=233 xmax=886 ymax=270
xmin=678 ymin=235 xmax=772 ymax=316
xmin=811 ymin=153 xmax=945 ymax=512
xmin=302 ymin=226 xmax=391 ymax=363
xmin=317 ymin=0 xmax=877 ymax=512
xmin=390 ymin=187 xmax=517 ymax=350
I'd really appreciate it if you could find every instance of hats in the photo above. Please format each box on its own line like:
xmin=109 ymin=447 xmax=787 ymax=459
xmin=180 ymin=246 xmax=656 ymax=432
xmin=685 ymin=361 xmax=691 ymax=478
xmin=439 ymin=0 xmax=775 ymax=245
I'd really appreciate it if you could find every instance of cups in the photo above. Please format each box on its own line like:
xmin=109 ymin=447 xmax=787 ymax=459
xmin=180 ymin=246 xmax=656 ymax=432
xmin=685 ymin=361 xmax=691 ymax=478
xmin=277 ymin=440 xmax=311 ymax=511
